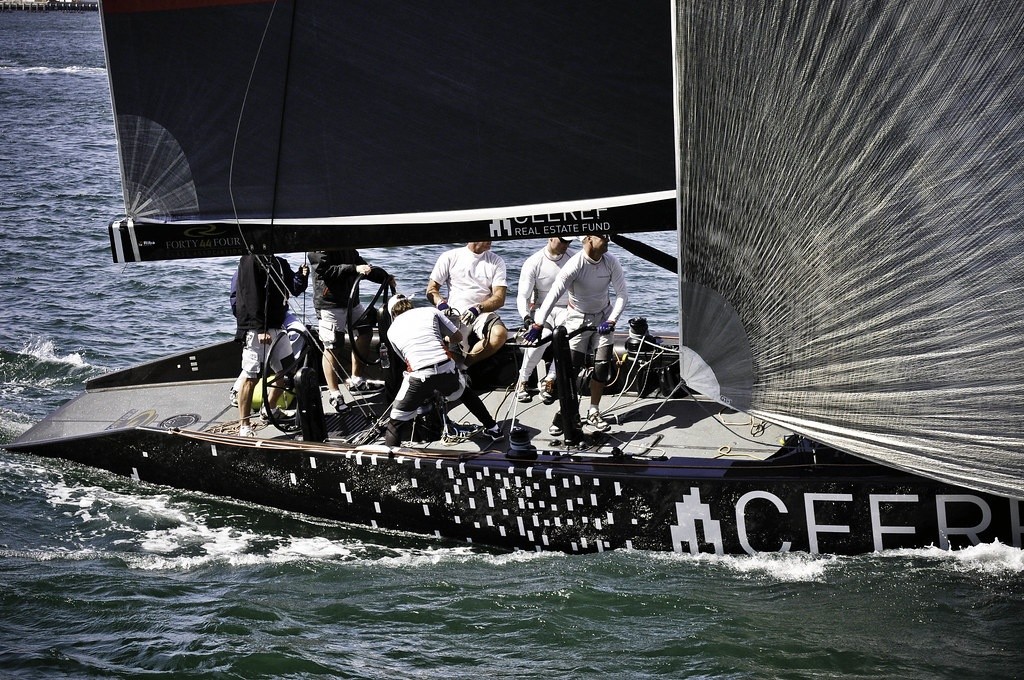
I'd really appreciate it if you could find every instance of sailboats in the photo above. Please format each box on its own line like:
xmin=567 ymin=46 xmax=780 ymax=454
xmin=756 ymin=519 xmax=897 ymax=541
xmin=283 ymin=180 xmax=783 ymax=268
xmin=0 ymin=0 xmax=1024 ymax=555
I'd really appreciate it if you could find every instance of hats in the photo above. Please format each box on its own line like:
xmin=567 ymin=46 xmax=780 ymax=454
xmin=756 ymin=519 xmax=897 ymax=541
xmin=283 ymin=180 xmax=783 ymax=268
xmin=562 ymin=236 xmax=578 ymax=240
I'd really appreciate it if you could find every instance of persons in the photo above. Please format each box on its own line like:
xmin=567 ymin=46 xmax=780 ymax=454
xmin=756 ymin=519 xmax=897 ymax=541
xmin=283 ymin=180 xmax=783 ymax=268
xmin=231 ymin=252 xmax=311 ymax=435
xmin=521 ymin=236 xmax=629 ymax=434
xmin=514 ymin=237 xmax=578 ymax=404
xmin=426 ymin=241 xmax=508 ymax=387
xmin=384 ymin=294 xmax=504 ymax=447
xmin=307 ymin=249 xmax=396 ymax=411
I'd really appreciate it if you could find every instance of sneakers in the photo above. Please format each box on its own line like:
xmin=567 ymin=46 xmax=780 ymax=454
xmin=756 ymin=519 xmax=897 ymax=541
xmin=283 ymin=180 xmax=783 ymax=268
xmin=239 ymin=428 xmax=257 ymax=438
xmin=537 ymin=375 xmax=555 ymax=404
xmin=329 ymin=395 xmax=351 ymax=414
xmin=229 ymin=386 xmax=239 ymax=406
xmin=549 ymin=424 xmax=562 ymax=435
xmin=482 ymin=427 xmax=504 ymax=441
xmin=514 ymin=380 xmax=531 ymax=401
xmin=348 ymin=380 xmax=384 ymax=396
xmin=260 ymin=405 xmax=296 ymax=422
xmin=586 ymin=410 xmax=611 ymax=431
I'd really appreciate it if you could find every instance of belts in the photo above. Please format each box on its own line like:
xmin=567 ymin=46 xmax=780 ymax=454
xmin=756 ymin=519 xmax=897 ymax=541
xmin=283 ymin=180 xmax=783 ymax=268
xmin=418 ymin=359 xmax=450 ymax=371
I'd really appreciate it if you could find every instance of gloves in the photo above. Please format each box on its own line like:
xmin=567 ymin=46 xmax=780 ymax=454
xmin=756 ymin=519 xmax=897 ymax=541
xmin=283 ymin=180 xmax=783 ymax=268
xmin=356 ymin=264 xmax=371 ymax=275
xmin=461 ymin=306 xmax=481 ymax=326
xmin=597 ymin=320 xmax=616 ymax=335
xmin=524 ymin=316 xmax=534 ymax=331
xmin=521 ymin=324 xmax=544 ymax=346
xmin=437 ymin=300 xmax=451 ymax=317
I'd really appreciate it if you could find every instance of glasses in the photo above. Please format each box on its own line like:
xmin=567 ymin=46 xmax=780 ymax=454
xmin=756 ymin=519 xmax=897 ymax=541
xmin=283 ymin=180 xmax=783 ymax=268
xmin=559 ymin=236 xmax=572 ymax=245
xmin=597 ymin=234 xmax=610 ymax=241
